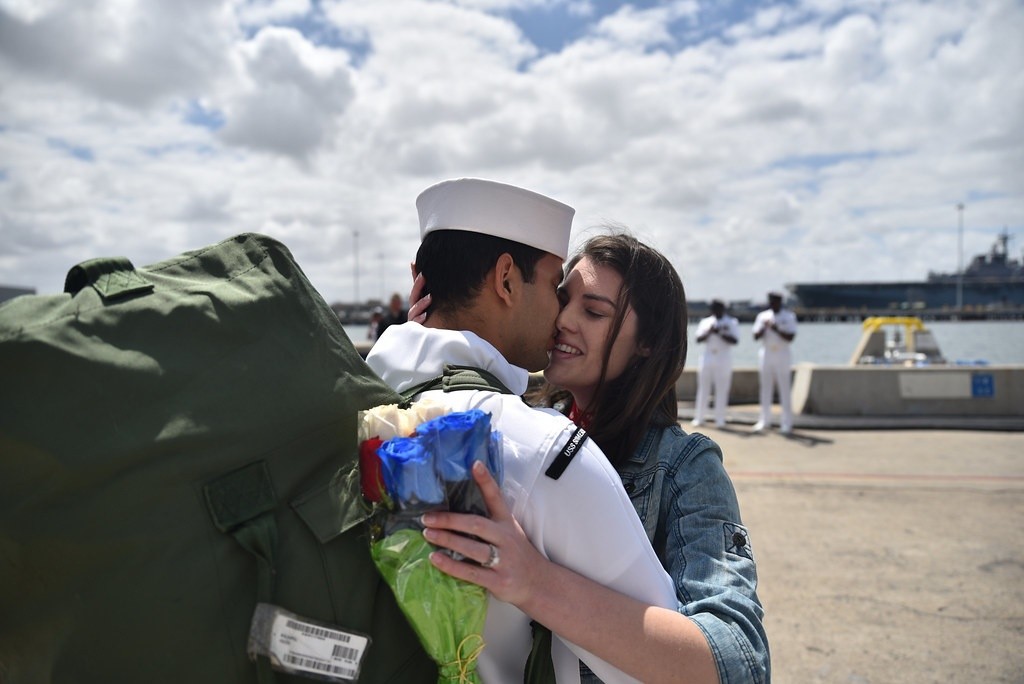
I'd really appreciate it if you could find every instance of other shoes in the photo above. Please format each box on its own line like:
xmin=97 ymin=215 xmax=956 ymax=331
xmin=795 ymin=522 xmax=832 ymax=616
xmin=779 ymin=425 xmax=793 ymax=435
xmin=752 ymin=421 xmax=771 ymax=432
xmin=715 ymin=419 xmax=725 ymax=430
xmin=690 ymin=419 xmax=704 ymax=427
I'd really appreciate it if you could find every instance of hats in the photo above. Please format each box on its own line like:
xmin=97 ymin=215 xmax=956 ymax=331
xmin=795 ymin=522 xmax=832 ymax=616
xmin=767 ymin=287 xmax=788 ymax=304
xmin=416 ymin=177 xmax=576 ymax=264
xmin=710 ymin=294 xmax=730 ymax=307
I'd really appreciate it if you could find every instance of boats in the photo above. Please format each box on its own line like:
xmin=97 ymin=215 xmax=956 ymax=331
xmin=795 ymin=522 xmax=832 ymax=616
xmin=848 ymin=316 xmax=990 ymax=365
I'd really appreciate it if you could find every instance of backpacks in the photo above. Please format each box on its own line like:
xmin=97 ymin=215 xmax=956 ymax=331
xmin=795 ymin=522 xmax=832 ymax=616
xmin=0 ymin=232 xmax=558 ymax=684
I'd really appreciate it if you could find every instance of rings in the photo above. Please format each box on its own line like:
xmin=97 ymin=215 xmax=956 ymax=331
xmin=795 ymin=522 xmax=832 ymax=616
xmin=482 ymin=545 xmax=499 ymax=566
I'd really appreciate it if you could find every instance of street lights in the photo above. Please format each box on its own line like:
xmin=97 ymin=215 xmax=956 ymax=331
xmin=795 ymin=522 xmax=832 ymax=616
xmin=956 ymin=200 xmax=967 ymax=319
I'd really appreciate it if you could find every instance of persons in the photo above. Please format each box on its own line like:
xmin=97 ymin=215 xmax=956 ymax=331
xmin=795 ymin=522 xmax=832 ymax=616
xmin=690 ymin=299 xmax=739 ymax=427
xmin=353 ymin=179 xmax=683 ymax=684
xmin=366 ymin=294 xmax=408 ymax=341
xmin=752 ymin=292 xmax=796 ymax=434
xmin=407 ymin=235 xmax=772 ymax=684
